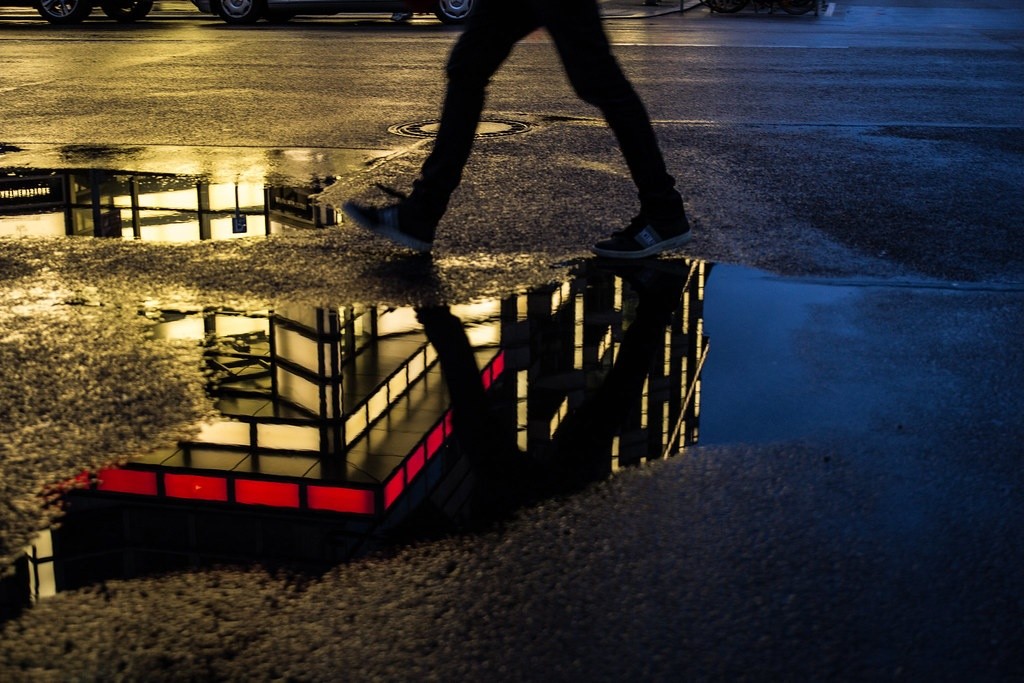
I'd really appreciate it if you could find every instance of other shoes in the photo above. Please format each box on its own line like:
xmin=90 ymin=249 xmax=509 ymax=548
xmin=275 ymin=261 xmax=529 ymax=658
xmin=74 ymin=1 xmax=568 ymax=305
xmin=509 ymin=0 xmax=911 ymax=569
xmin=590 ymin=191 xmax=693 ymax=260
xmin=344 ymin=179 xmax=452 ymax=252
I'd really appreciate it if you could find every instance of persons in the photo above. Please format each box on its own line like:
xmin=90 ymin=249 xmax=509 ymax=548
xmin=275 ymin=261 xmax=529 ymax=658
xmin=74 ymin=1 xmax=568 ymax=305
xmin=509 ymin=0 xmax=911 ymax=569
xmin=344 ymin=0 xmax=692 ymax=258
